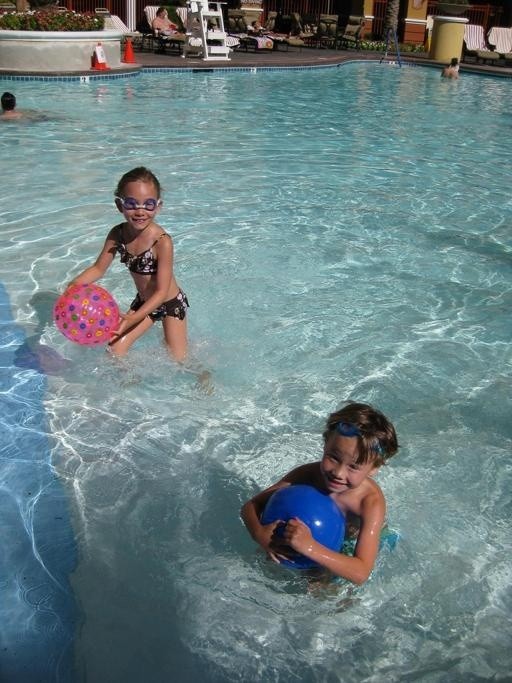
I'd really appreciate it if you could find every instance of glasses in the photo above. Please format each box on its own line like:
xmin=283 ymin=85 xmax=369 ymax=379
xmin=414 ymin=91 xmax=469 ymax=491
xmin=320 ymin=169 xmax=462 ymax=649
xmin=337 ymin=421 xmax=384 ymax=456
xmin=118 ymin=198 xmax=161 ymax=211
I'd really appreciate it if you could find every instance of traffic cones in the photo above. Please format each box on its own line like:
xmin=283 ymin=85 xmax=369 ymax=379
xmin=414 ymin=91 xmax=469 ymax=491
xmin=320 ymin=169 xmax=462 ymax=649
xmin=122 ymin=36 xmax=137 ymax=63
xmin=91 ymin=41 xmax=111 ymax=70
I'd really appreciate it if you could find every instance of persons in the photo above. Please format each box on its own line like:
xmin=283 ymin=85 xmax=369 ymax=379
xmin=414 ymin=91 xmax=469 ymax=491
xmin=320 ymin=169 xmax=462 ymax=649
xmin=242 ymin=400 xmax=400 ymax=587
xmin=441 ymin=57 xmax=459 ymax=80
xmin=63 ymin=165 xmax=214 ymax=392
xmin=252 ymin=19 xmax=303 ymax=39
xmin=0 ymin=90 xmax=26 ymax=121
xmin=153 ymin=6 xmax=191 ymax=40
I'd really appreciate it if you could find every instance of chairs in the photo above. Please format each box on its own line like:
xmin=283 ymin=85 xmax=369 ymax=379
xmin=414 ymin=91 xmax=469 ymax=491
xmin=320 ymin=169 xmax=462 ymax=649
xmin=95 ymin=5 xmax=367 ymax=54
xmin=487 ymin=26 xmax=511 ymax=60
xmin=463 ymin=23 xmax=500 ymax=65
